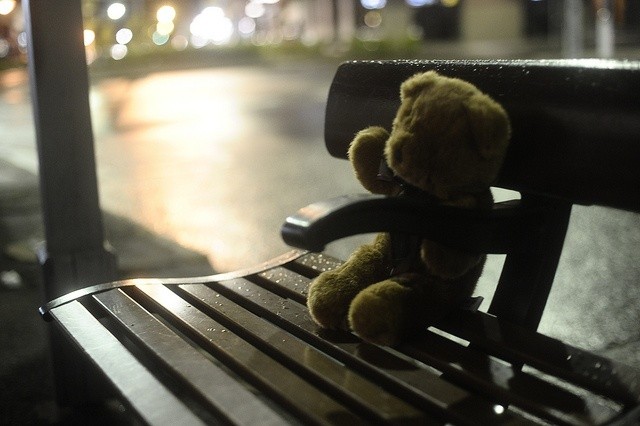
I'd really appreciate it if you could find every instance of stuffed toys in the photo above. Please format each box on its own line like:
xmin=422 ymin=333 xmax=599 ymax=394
xmin=306 ymin=70 xmax=511 ymax=346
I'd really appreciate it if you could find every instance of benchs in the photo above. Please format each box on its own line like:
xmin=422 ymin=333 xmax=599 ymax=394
xmin=37 ymin=58 xmax=640 ymax=426
